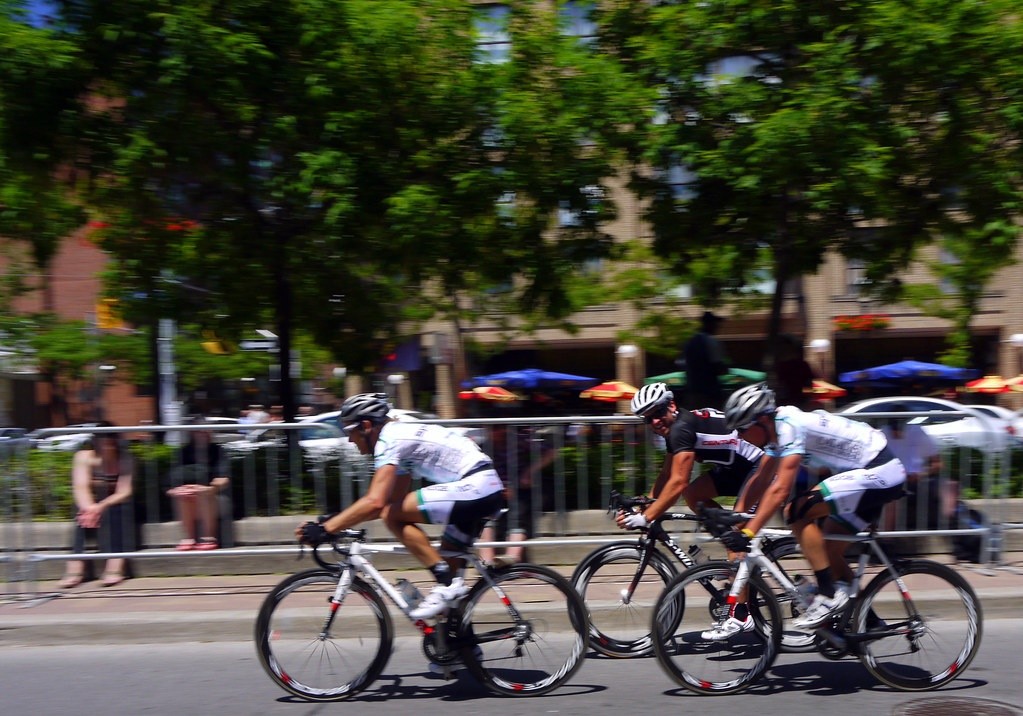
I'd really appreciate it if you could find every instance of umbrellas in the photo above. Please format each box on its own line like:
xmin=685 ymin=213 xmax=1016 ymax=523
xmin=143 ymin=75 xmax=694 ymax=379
xmin=456 ymin=360 xmax=1023 ymax=413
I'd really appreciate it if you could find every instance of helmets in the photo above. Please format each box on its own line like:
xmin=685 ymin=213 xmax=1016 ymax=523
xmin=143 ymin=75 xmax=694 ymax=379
xmin=630 ymin=382 xmax=674 ymax=416
xmin=724 ymin=381 xmax=779 ymax=429
xmin=340 ymin=393 xmax=390 ymax=422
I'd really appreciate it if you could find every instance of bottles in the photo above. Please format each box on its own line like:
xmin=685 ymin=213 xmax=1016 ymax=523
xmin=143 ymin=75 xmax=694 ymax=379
xmin=793 ymin=574 xmax=818 ymax=604
xmin=687 ymin=544 xmax=730 ymax=592
xmin=396 ymin=578 xmax=438 ymax=628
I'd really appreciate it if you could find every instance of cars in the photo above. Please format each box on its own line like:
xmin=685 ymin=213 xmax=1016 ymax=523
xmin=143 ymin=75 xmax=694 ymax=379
xmin=0 ymin=397 xmax=1023 ymax=459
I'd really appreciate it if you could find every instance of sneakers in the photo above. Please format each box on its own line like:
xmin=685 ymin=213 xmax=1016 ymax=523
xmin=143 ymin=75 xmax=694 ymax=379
xmin=409 ymin=577 xmax=472 ymax=620
xmin=429 ymin=645 xmax=483 ymax=674
xmin=701 ymin=613 xmax=756 ymax=641
xmin=795 ymin=589 xmax=851 ymax=628
xmin=864 ymin=620 xmax=889 ymax=647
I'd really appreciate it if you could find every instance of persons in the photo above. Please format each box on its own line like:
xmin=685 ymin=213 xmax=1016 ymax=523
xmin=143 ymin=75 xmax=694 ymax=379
xmin=478 ymin=423 xmax=560 ymax=570
xmin=722 ymin=381 xmax=907 ymax=628
xmin=522 ymin=421 xmax=616 ymax=507
xmin=858 ymin=405 xmax=943 ymax=531
xmin=159 ymin=415 xmax=233 ymax=551
xmin=294 ymin=403 xmax=344 ymax=439
xmin=615 ymin=382 xmax=811 ymax=642
xmin=682 ymin=310 xmax=730 ymax=382
xmin=293 ymin=391 xmax=511 ymax=673
xmin=56 ymin=421 xmax=137 ymax=588
xmin=237 ymin=404 xmax=287 ymax=440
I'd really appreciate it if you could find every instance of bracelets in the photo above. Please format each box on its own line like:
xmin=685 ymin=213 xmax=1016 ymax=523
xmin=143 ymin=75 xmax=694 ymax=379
xmin=741 ymin=529 xmax=755 ymax=539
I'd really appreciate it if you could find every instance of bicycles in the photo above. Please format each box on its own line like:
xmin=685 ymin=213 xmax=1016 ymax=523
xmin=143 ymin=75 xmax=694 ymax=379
xmin=650 ymin=500 xmax=983 ymax=695
xmin=253 ymin=527 xmax=588 ymax=703
xmin=566 ymin=490 xmax=825 ymax=659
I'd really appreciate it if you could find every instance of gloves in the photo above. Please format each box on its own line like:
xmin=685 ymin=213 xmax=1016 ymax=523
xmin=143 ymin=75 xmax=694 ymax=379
xmin=720 ymin=526 xmax=754 ymax=552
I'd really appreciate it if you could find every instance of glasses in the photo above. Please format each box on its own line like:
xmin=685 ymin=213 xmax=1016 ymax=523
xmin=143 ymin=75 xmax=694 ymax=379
xmin=643 ymin=402 xmax=669 ymax=424
xmin=343 ymin=421 xmax=361 ymax=437
xmin=736 ymin=419 xmax=758 ymax=434
